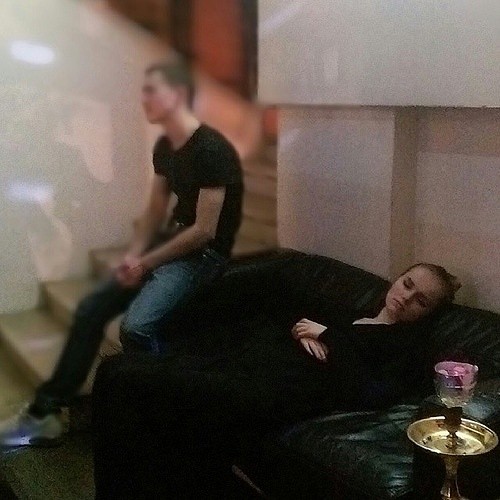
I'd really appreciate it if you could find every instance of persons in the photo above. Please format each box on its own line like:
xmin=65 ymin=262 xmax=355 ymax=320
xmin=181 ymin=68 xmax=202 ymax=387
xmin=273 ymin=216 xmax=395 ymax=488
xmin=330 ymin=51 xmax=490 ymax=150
xmin=1 ymin=57 xmax=249 ymax=451
xmin=87 ymin=262 xmax=465 ymax=500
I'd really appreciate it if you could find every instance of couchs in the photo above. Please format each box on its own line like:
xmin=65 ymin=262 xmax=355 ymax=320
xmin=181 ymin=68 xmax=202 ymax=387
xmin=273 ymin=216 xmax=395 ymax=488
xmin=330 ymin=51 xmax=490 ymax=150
xmin=93 ymin=248 xmax=500 ymax=500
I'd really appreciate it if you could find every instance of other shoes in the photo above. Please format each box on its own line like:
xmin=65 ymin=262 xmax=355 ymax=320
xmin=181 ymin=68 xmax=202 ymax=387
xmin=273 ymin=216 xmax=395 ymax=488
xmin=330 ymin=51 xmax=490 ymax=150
xmin=0 ymin=403 xmax=64 ymax=446
